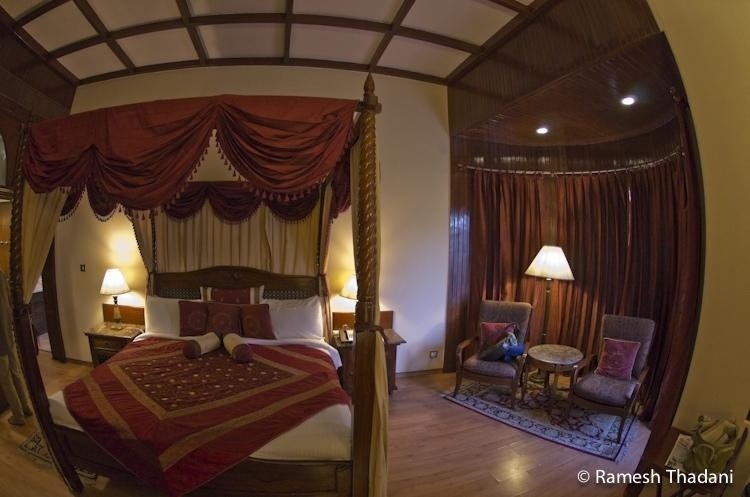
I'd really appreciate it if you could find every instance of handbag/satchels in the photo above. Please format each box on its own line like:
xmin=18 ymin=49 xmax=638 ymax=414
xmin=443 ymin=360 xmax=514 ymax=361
xmin=684 ymin=414 xmax=738 ymax=482
xmin=478 ymin=334 xmax=519 ymax=360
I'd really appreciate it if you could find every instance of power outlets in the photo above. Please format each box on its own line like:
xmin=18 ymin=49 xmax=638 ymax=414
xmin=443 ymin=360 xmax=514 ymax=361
xmin=430 ymin=351 xmax=438 ymax=358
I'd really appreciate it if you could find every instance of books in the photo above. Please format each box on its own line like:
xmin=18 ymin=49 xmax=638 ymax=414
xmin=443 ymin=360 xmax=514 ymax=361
xmin=666 ymin=433 xmax=693 ymax=477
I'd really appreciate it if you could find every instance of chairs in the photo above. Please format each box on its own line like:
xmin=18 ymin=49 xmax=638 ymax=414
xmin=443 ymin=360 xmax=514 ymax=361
xmin=454 ymin=301 xmax=655 ymax=445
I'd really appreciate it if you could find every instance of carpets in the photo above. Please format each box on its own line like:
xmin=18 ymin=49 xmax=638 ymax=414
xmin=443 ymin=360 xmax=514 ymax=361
xmin=14 ymin=428 xmax=97 ymax=490
xmin=440 ymin=378 xmax=640 ymax=464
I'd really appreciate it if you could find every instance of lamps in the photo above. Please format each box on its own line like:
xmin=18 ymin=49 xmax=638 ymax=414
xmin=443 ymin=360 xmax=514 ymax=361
xmin=338 ymin=274 xmax=356 ymax=300
xmin=524 ymin=246 xmax=575 ymax=345
xmin=99 ymin=269 xmax=131 ymax=330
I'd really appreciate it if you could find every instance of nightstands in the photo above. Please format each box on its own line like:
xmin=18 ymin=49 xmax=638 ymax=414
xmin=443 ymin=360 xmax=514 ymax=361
xmin=330 ymin=310 xmax=404 ymax=394
xmin=82 ymin=319 xmax=144 ymax=366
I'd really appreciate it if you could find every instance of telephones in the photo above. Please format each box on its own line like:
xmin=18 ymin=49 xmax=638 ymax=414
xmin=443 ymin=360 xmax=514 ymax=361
xmin=339 ymin=325 xmax=354 ymax=343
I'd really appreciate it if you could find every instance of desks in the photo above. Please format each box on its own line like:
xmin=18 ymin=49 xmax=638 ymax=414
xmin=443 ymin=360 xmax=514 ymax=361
xmin=656 ymin=426 xmax=729 ymax=497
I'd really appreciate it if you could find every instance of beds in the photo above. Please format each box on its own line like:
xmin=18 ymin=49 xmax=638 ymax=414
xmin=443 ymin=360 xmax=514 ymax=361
xmin=48 ymin=264 xmax=357 ymax=497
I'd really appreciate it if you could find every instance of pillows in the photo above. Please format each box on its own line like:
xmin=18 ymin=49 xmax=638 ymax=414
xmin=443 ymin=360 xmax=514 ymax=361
xmin=143 ymin=286 xmax=323 ymax=361
